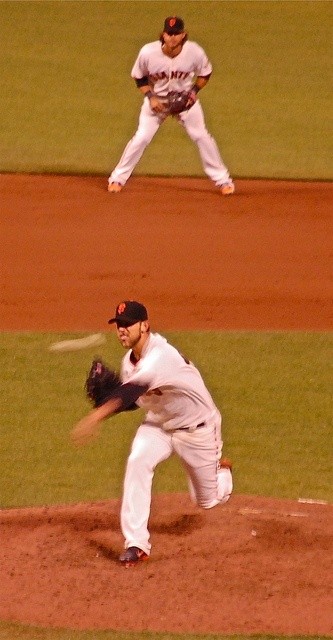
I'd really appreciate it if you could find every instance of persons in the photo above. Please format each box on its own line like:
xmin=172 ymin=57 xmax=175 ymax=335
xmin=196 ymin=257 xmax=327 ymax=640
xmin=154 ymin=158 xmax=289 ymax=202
xmin=108 ymin=15 xmax=236 ymax=194
xmin=72 ymin=298 xmax=233 ymax=564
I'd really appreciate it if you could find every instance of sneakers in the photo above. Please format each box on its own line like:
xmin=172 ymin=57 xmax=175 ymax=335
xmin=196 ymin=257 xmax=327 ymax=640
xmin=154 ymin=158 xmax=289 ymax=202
xmin=118 ymin=547 xmax=149 ymax=569
xmin=107 ymin=181 xmax=123 ymax=193
xmin=218 ymin=458 xmax=233 ymax=473
xmin=220 ymin=182 xmax=235 ymax=196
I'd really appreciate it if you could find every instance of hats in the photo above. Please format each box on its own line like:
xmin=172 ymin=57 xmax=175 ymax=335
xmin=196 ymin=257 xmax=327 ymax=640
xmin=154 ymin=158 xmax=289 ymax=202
xmin=163 ymin=17 xmax=183 ymax=32
xmin=108 ymin=301 xmax=148 ymax=324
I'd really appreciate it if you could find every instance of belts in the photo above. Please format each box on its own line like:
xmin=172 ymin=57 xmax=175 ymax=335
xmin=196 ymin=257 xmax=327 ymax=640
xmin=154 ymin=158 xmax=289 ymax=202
xmin=177 ymin=421 xmax=206 ymax=432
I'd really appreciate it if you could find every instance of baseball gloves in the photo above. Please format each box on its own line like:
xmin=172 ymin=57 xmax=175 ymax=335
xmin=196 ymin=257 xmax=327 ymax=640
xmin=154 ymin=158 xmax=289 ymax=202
xmin=82 ymin=356 xmax=127 ymax=419
xmin=164 ymin=86 xmax=198 ymax=116
